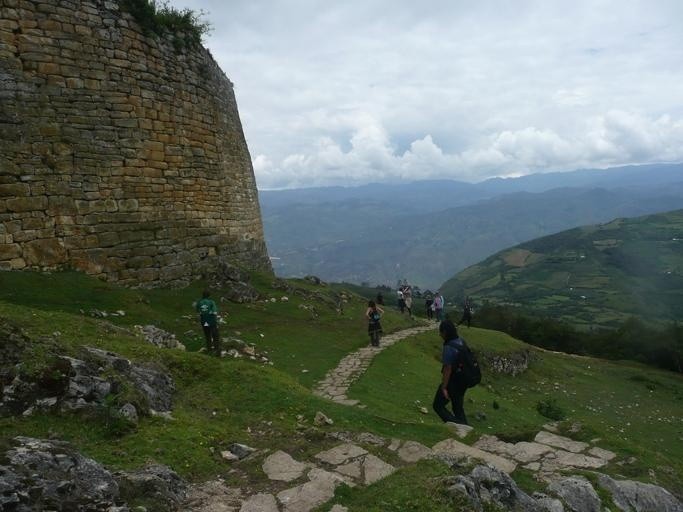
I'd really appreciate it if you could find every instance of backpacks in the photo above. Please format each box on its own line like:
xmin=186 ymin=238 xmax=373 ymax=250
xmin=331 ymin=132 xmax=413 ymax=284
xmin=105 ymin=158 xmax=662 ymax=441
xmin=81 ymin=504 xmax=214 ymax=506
xmin=370 ymin=310 xmax=381 ymax=321
xmin=442 ymin=337 xmax=482 ymax=389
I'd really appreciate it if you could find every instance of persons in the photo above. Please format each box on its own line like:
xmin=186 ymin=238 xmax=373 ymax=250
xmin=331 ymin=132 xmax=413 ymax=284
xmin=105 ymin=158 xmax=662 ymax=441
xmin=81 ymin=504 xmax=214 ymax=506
xmin=365 ymin=302 xmax=385 ymax=348
xmin=193 ymin=291 xmax=222 ymax=358
xmin=375 ymin=291 xmax=385 ymax=306
xmin=455 ymin=297 xmax=473 ymax=328
xmin=395 ymin=285 xmax=444 ymax=324
xmin=430 ymin=319 xmax=471 ymax=427
